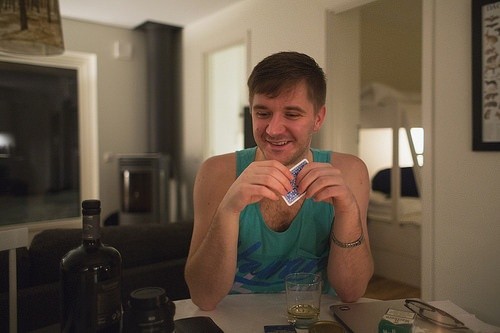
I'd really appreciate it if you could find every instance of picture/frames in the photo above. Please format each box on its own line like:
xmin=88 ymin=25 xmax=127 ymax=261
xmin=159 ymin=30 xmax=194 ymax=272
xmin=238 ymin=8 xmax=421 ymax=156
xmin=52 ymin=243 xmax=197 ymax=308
xmin=470 ymin=0 xmax=500 ymax=151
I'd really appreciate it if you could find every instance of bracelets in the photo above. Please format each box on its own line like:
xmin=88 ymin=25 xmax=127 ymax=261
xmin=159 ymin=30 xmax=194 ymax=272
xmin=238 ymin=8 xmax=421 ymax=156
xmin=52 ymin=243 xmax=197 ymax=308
xmin=329 ymin=229 xmax=364 ymax=249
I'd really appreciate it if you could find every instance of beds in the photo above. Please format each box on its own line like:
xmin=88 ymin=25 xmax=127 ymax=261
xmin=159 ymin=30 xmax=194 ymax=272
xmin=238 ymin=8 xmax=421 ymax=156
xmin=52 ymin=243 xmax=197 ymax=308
xmin=359 ymin=103 xmax=424 ymax=288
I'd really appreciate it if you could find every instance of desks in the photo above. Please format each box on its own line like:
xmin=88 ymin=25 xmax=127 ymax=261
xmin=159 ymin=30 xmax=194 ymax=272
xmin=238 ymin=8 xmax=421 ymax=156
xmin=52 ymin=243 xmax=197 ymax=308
xmin=172 ymin=292 xmax=383 ymax=333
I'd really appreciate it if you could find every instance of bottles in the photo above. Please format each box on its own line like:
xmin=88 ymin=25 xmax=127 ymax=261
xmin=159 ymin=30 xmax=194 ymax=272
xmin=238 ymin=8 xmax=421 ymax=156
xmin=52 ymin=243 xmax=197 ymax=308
xmin=61 ymin=200 xmax=122 ymax=333
xmin=131 ymin=288 xmax=176 ymax=333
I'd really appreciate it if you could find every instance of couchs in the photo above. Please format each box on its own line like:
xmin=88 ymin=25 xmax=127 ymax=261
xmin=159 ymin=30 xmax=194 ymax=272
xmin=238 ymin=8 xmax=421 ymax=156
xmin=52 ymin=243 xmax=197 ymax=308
xmin=0 ymin=219 xmax=194 ymax=333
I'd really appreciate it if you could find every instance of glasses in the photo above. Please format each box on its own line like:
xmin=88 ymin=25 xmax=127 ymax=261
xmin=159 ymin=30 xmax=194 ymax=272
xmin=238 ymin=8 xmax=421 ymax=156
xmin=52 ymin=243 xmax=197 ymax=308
xmin=404 ymin=299 xmax=469 ymax=329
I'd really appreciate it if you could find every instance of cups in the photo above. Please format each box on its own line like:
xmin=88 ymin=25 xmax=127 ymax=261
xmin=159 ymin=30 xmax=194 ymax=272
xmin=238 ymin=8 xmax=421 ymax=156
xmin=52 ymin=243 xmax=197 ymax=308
xmin=284 ymin=272 xmax=322 ymax=329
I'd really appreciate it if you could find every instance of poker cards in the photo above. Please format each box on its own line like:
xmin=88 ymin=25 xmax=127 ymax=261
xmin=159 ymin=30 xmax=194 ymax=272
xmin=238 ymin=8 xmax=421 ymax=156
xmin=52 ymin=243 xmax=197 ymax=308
xmin=277 ymin=159 xmax=320 ymax=207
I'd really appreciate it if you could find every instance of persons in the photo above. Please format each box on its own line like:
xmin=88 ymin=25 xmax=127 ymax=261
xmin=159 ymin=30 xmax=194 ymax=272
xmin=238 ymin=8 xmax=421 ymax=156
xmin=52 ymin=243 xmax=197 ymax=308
xmin=185 ymin=50 xmax=375 ymax=311
